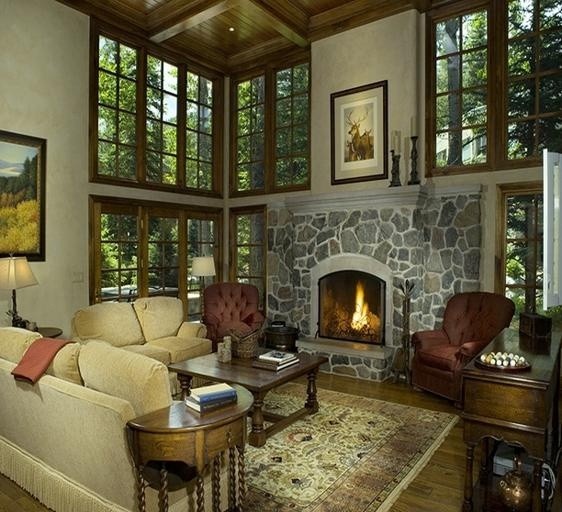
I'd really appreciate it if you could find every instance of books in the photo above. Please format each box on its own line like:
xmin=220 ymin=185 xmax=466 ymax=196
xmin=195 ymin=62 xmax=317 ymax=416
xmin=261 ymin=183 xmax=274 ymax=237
xmin=252 ymin=350 xmax=299 ymax=372
xmin=190 ymin=382 xmax=237 ymax=401
xmin=185 ymin=396 xmax=238 ymax=412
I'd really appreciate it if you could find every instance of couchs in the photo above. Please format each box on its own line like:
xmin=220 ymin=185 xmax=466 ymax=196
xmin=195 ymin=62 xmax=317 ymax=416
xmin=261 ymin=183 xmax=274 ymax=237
xmin=1 ymin=326 xmax=239 ymax=512
xmin=73 ymin=296 xmax=216 ymax=394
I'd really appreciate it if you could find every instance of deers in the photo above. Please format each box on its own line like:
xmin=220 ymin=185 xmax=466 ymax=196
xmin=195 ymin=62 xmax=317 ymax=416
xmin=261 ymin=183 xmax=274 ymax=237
xmin=345 ymin=109 xmax=375 ymax=162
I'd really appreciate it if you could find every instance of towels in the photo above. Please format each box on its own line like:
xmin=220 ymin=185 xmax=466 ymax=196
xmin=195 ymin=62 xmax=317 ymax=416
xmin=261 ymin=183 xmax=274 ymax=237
xmin=10 ymin=337 xmax=77 ymax=386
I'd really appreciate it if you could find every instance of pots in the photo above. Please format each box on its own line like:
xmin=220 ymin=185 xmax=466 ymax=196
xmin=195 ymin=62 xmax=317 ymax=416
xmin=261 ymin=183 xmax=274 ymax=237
xmin=264 ymin=321 xmax=300 ymax=350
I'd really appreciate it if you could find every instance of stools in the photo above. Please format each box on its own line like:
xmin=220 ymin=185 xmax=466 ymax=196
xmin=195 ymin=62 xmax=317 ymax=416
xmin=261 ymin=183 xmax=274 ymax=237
xmin=264 ymin=327 xmax=300 ymax=352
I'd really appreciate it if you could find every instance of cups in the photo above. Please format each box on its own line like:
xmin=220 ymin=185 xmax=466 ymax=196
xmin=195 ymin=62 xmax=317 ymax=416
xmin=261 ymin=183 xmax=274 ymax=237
xmin=27 ymin=322 xmax=37 ymax=332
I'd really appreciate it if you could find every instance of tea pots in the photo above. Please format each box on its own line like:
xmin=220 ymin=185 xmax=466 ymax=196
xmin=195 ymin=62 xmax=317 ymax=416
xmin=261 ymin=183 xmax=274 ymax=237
xmin=499 ymin=456 xmax=532 ymax=510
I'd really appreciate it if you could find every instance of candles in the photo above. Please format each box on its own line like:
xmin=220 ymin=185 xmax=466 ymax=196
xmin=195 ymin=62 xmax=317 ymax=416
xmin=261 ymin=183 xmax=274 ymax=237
xmin=409 ymin=116 xmax=417 ymax=137
xmin=391 ymin=130 xmax=397 ymax=151
xmin=393 ymin=137 xmax=401 ymax=155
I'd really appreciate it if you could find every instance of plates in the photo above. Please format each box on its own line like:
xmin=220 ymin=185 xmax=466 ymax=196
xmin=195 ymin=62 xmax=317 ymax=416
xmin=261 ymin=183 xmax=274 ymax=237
xmin=475 ymin=354 xmax=530 ymax=371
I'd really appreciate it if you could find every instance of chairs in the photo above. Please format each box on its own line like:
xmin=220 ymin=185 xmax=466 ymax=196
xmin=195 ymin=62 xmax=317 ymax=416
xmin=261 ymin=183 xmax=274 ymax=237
xmin=202 ymin=283 xmax=264 ymax=343
xmin=409 ymin=292 xmax=517 ymax=409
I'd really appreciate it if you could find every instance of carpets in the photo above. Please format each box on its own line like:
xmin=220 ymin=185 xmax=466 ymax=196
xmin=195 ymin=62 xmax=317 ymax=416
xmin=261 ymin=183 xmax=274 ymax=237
xmin=172 ymin=378 xmax=458 ymax=511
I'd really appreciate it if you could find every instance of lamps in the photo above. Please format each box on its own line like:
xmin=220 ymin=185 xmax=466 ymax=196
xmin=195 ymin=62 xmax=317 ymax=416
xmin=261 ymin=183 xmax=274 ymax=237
xmin=189 ymin=256 xmax=217 ymax=318
xmin=0 ymin=257 xmax=40 ymax=326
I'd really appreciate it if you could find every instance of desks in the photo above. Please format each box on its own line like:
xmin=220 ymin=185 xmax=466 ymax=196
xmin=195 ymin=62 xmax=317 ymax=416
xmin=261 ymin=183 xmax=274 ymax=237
xmin=168 ymin=344 xmax=330 ymax=447
xmin=459 ymin=328 xmax=561 ymax=511
xmin=33 ymin=326 xmax=63 ymax=338
xmin=126 ymin=382 xmax=254 ymax=512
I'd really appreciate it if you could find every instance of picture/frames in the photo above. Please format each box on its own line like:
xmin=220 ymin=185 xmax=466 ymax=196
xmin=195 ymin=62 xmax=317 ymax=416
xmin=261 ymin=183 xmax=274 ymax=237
xmin=328 ymin=81 xmax=389 ymax=183
xmin=0 ymin=129 xmax=48 ymax=262
